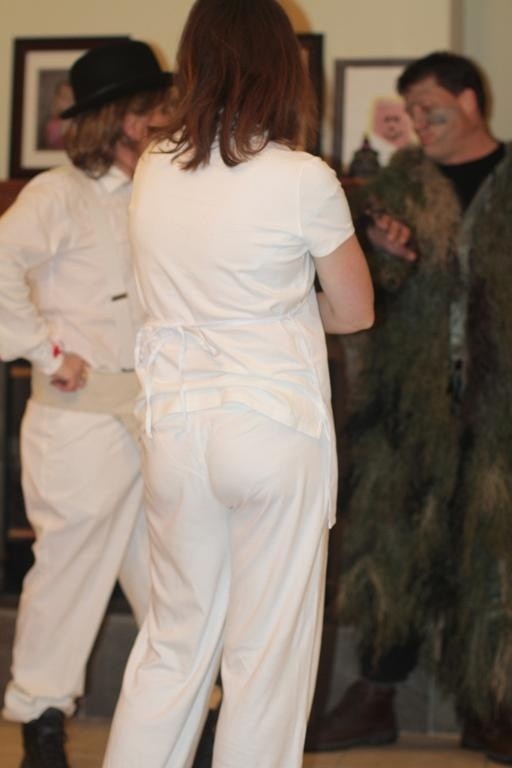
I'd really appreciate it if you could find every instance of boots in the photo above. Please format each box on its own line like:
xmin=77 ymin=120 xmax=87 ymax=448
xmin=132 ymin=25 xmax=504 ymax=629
xmin=304 ymin=686 xmax=397 ymax=751
xmin=459 ymin=707 xmax=512 ymax=765
xmin=16 ymin=707 xmax=69 ymax=768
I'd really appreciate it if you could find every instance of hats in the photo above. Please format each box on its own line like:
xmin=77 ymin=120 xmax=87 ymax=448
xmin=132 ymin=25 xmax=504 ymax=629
xmin=56 ymin=39 xmax=177 ymax=121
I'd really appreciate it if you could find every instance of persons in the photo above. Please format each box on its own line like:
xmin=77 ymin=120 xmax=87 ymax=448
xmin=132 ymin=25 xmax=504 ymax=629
xmin=343 ymin=89 xmax=422 ymax=184
xmin=306 ymin=50 xmax=511 ymax=768
xmin=94 ymin=1 xmax=381 ymax=767
xmin=0 ymin=33 xmax=176 ymax=768
xmin=37 ymin=80 xmax=76 ymax=146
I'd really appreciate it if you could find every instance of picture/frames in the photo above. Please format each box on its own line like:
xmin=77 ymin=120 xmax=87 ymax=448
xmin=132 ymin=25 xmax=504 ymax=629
xmin=290 ymin=32 xmax=418 ymax=180
xmin=7 ymin=36 xmax=139 ymax=180
